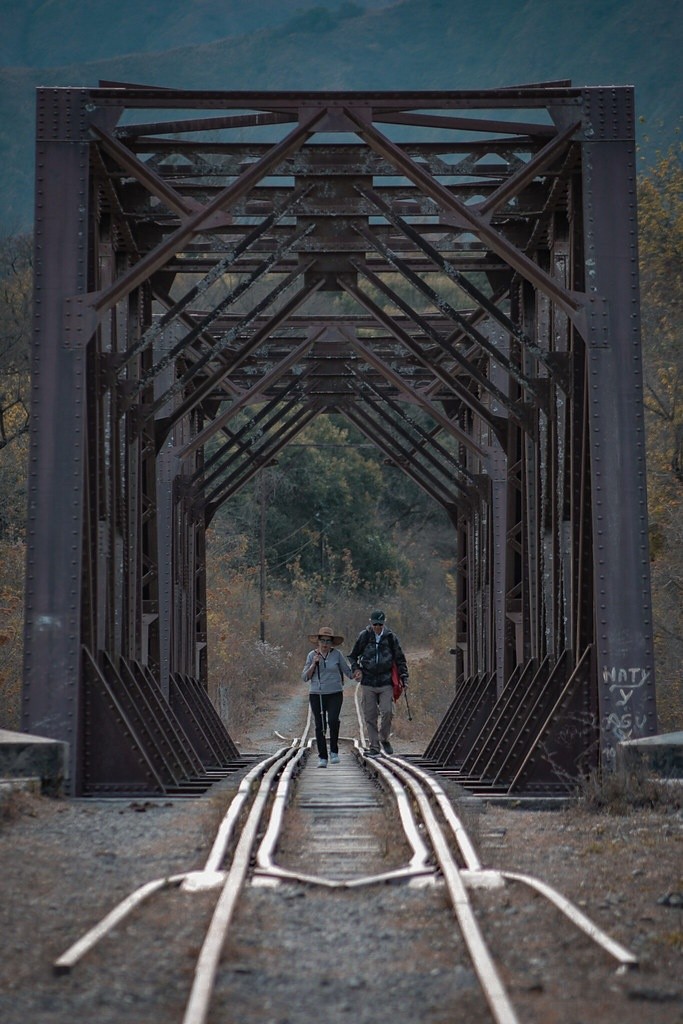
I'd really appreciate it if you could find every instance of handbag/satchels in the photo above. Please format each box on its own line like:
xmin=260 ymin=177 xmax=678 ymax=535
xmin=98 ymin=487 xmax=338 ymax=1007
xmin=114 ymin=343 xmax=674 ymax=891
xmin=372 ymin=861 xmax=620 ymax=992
xmin=392 ymin=660 xmax=403 ymax=704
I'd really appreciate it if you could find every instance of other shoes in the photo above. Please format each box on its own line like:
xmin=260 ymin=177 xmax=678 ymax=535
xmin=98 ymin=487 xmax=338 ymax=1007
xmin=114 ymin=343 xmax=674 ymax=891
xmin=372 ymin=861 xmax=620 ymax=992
xmin=330 ymin=752 xmax=340 ymax=764
xmin=318 ymin=759 xmax=328 ymax=768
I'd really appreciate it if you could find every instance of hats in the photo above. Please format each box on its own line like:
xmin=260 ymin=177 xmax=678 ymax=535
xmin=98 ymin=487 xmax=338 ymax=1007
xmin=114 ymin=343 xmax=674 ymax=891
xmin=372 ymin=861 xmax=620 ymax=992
xmin=371 ymin=610 xmax=385 ymax=625
xmin=308 ymin=627 xmax=344 ymax=647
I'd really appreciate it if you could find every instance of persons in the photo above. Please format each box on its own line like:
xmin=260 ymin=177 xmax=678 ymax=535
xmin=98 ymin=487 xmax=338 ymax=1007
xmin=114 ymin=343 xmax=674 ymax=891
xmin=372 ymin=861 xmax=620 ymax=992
xmin=301 ymin=627 xmax=362 ymax=768
xmin=346 ymin=610 xmax=409 ymax=757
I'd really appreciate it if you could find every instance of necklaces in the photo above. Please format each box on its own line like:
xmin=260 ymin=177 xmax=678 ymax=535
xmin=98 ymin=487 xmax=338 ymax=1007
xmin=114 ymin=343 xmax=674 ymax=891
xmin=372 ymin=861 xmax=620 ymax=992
xmin=319 ymin=649 xmax=331 ymax=662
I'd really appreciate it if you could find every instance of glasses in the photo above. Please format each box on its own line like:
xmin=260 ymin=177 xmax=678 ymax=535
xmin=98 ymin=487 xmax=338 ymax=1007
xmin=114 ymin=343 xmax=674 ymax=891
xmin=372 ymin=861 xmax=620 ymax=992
xmin=373 ymin=623 xmax=383 ymax=626
xmin=319 ymin=639 xmax=332 ymax=644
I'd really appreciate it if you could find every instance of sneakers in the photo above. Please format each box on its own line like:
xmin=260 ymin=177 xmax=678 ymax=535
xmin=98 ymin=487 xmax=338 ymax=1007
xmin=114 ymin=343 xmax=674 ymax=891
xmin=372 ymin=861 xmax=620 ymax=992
xmin=363 ymin=749 xmax=381 ymax=758
xmin=381 ymin=742 xmax=393 ymax=754
